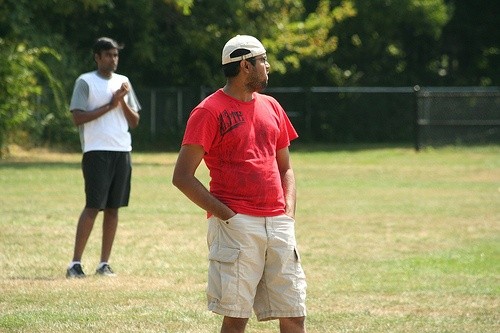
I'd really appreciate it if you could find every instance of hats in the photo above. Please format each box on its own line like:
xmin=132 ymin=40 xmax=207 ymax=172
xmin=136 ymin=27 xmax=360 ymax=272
xmin=221 ymin=34 xmax=266 ymax=65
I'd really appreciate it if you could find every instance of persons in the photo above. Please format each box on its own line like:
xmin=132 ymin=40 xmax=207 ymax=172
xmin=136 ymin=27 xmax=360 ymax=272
xmin=66 ymin=36 xmax=143 ymax=279
xmin=172 ymin=34 xmax=307 ymax=333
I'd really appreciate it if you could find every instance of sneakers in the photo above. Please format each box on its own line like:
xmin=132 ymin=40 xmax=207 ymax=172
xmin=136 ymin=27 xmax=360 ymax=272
xmin=65 ymin=264 xmax=86 ymax=279
xmin=95 ymin=264 xmax=117 ymax=278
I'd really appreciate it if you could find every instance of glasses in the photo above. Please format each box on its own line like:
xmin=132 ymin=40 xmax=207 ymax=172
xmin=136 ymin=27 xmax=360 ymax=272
xmin=250 ymin=55 xmax=268 ymax=63
